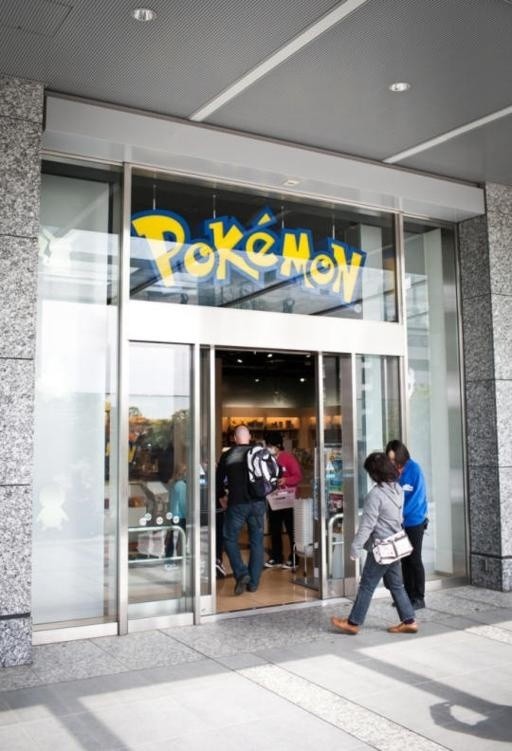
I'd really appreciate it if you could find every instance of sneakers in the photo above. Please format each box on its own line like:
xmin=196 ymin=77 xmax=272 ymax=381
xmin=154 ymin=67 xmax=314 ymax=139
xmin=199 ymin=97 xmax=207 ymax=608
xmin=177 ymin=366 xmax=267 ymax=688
xmin=214 ymin=559 xmax=228 ymax=577
xmin=264 ymin=557 xmax=298 ymax=572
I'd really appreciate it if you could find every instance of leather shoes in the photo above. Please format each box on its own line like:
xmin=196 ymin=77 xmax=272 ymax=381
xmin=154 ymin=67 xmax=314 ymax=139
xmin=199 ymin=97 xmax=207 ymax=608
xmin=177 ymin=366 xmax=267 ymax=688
xmin=392 ymin=597 xmax=425 ymax=611
xmin=331 ymin=615 xmax=359 ymax=631
xmin=389 ymin=623 xmax=418 ymax=632
xmin=234 ymin=573 xmax=258 ymax=594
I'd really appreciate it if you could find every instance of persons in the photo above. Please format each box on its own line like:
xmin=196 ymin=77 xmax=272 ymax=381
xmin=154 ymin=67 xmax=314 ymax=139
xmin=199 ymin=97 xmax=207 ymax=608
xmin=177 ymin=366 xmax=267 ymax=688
xmin=327 ymin=450 xmax=418 ymax=638
xmin=383 ymin=437 xmax=432 ymax=611
xmin=216 ymin=459 xmax=227 ymax=579
xmin=164 ymin=464 xmax=187 ymax=571
xmin=262 ymin=430 xmax=304 ymax=570
xmin=218 ymin=424 xmax=280 ymax=596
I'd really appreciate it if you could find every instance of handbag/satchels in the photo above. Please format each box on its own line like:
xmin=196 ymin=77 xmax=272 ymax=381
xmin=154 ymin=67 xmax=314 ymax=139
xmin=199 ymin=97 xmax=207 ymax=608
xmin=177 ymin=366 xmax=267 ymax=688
xmin=372 ymin=531 xmax=414 ymax=566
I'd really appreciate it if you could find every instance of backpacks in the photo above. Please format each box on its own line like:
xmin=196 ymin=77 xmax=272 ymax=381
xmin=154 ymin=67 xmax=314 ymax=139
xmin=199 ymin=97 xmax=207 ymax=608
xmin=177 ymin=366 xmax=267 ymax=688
xmin=246 ymin=446 xmax=280 ymax=498
